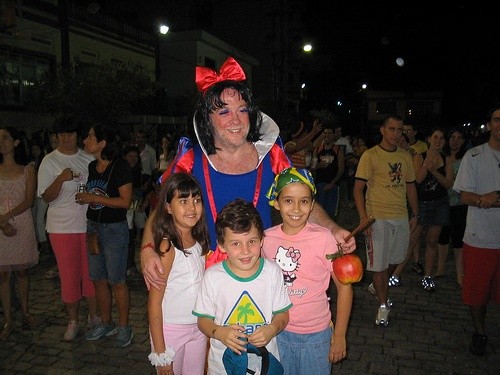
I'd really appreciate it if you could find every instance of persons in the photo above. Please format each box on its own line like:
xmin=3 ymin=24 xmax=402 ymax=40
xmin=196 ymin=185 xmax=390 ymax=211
xmin=75 ymin=123 xmax=135 ymax=347
xmin=259 ymin=167 xmax=353 ymax=375
xmin=0 ymin=126 xmax=40 ymax=341
xmin=353 ymin=113 xmax=418 ymax=327
xmin=389 ymin=119 xmax=472 ymax=291
xmin=148 ymin=172 xmax=214 ymax=375
xmin=140 ymin=56 xmax=357 ymax=292
xmin=453 ymin=104 xmax=500 ymax=358
xmin=192 ymin=199 xmax=293 ymax=375
xmin=282 ymin=119 xmax=369 ymax=222
xmin=30 ymin=129 xmax=181 ymax=278
xmin=36 ymin=119 xmax=97 ymax=342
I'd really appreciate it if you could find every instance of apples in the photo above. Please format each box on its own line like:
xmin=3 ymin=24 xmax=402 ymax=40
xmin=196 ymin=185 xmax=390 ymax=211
xmin=332 ymin=253 xmax=363 ymax=284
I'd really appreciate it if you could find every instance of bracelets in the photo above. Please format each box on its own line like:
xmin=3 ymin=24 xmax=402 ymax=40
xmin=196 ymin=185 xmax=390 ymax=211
xmin=140 ymin=244 xmax=154 ymax=251
xmin=9 ymin=209 xmax=15 ymax=218
xmin=413 ymin=214 xmax=420 ymax=219
xmin=148 ymin=346 xmax=175 ymax=367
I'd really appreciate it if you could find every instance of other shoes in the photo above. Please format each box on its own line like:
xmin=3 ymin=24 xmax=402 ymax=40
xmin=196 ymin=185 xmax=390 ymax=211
xmin=44 ymin=270 xmax=59 ymax=278
xmin=469 ymin=334 xmax=487 ymax=355
xmin=22 ymin=315 xmax=43 ymax=329
xmin=413 ymin=261 xmax=424 ymax=273
xmin=0 ymin=321 xmax=13 ymax=342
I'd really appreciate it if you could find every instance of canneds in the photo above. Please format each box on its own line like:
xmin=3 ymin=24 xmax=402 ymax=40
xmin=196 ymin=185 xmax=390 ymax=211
xmin=312 ymin=156 xmax=318 ymax=168
xmin=78 ymin=184 xmax=85 ymax=193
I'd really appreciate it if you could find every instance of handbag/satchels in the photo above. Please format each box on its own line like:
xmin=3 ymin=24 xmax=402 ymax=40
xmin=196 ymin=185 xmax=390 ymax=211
xmin=135 ymin=198 xmax=146 ymax=228
xmin=88 ymin=231 xmax=101 ymax=255
xmin=126 ymin=202 xmax=133 ymax=229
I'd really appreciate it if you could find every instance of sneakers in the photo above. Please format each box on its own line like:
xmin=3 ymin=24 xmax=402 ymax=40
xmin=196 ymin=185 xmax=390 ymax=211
xmin=388 ymin=276 xmax=400 ymax=286
xmin=85 ymin=322 xmax=117 ymax=340
xmin=64 ymin=321 xmax=79 ymax=341
xmin=368 ymin=283 xmax=377 ymax=295
xmin=115 ymin=326 xmax=133 ymax=347
xmin=422 ymin=278 xmax=435 ymax=290
xmin=376 ymin=305 xmax=390 ymax=326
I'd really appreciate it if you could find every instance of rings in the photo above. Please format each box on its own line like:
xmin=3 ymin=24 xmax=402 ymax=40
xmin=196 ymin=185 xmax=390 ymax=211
xmin=79 ymin=198 xmax=82 ymax=201
